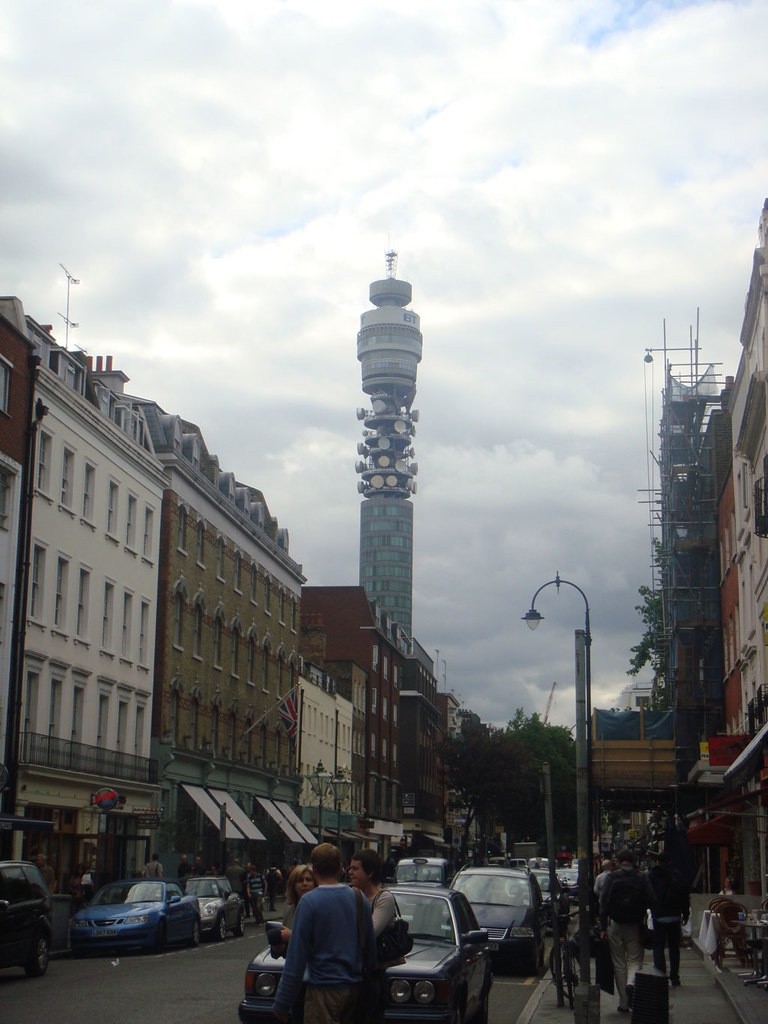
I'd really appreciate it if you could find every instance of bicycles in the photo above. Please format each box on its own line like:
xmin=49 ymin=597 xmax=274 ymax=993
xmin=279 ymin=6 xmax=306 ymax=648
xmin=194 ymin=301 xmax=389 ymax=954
xmin=540 ymin=908 xmax=581 ymax=1010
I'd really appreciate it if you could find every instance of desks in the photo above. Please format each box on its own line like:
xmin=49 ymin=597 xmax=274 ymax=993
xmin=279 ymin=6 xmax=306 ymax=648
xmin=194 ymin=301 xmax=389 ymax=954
xmin=699 ymin=909 xmax=768 ymax=991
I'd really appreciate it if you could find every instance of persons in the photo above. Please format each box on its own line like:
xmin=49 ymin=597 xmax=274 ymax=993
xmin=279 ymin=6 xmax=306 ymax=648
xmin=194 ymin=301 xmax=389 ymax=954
xmin=223 ymin=858 xmax=282 ymax=927
xmin=35 ymin=854 xmax=55 ymax=892
xmin=178 ymin=854 xmax=216 ymax=877
xmin=141 ymin=853 xmax=163 ymax=877
xmin=66 ymin=863 xmax=87 ymax=918
xmin=272 ymin=843 xmax=396 ymax=1024
xmin=593 ymin=849 xmax=690 ymax=1012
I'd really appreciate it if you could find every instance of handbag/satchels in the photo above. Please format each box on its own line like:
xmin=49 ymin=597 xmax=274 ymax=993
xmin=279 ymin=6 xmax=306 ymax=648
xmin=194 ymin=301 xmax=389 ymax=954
xmin=371 ymin=889 xmax=414 ymax=962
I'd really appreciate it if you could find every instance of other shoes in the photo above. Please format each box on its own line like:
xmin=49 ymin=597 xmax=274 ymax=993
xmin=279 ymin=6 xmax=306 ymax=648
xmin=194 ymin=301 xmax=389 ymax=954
xmin=672 ymin=980 xmax=680 ymax=986
xmin=617 ymin=1005 xmax=629 ymax=1014
xmin=625 ymin=985 xmax=635 ymax=1009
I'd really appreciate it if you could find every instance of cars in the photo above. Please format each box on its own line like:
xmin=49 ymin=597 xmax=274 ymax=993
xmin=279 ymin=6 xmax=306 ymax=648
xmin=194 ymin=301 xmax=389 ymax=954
xmin=238 ymin=881 xmax=493 ymax=1024
xmin=484 ymin=855 xmax=582 ymax=929
xmin=70 ymin=877 xmax=203 ymax=959
xmin=177 ymin=874 xmax=246 ymax=941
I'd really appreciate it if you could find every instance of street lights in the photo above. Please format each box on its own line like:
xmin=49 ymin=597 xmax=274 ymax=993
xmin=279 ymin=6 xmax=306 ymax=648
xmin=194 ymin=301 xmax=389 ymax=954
xmin=519 ymin=578 xmax=598 ymax=960
xmin=329 ymin=765 xmax=354 ymax=851
xmin=304 ymin=758 xmax=333 ymax=845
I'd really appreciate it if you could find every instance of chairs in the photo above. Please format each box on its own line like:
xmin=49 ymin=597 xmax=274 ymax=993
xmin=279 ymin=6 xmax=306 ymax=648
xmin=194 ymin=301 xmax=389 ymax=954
xmin=709 ymin=897 xmax=768 ymax=968
xmin=507 ymin=883 xmax=527 ymax=905
xmin=407 ymin=902 xmax=443 ymax=936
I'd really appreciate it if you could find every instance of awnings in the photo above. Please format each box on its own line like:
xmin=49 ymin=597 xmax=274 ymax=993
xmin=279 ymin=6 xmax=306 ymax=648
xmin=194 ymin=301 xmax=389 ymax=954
xmin=183 ymin=784 xmax=318 ymax=844
xmin=424 ymin=834 xmax=444 ymax=844
xmin=0 ymin=813 xmax=53 ymax=833
xmin=310 ymin=826 xmax=377 ymax=841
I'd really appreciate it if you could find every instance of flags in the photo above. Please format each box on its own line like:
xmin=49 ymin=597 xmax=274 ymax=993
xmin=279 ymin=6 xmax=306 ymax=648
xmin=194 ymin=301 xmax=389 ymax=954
xmin=277 ymin=688 xmax=297 ymax=754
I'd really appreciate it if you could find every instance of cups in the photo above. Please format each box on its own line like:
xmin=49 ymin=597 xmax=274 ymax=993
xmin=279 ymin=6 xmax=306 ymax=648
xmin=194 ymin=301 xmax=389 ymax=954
xmin=737 ymin=912 xmax=744 ymax=920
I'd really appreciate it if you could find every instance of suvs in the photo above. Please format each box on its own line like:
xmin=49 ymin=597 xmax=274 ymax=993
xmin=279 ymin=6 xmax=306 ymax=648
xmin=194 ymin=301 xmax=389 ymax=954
xmin=0 ymin=858 xmax=56 ymax=978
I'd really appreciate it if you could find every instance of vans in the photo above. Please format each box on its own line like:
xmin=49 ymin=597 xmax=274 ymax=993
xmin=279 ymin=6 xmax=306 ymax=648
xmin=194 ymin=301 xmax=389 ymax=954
xmin=393 ymin=856 xmax=448 ymax=905
xmin=446 ymin=861 xmax=549 ymax=976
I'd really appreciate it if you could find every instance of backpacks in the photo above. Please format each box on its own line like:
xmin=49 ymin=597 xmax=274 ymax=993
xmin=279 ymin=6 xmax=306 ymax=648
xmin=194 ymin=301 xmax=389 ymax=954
xmin=266 ymin=869 xmax=278 ymax=890
xmin=602 ymin=871 xmax=646 ymax=923
